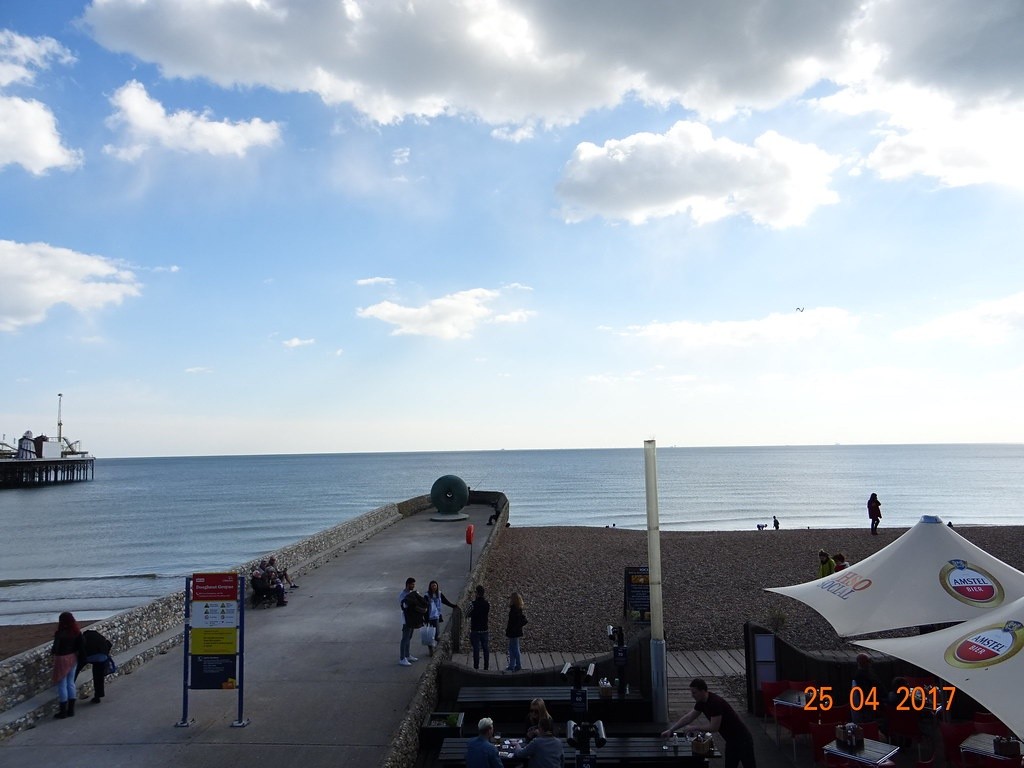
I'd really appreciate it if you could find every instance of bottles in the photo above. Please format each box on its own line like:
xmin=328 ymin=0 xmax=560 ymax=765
xmin=672 ymin=733 xmax=678 ymax=745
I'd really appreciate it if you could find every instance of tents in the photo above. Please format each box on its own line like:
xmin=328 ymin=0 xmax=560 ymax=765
xmin=762 ymin=515 xmax=1024 ymax=639
xmin=847 ymin=597 xmax=1024 ymax=744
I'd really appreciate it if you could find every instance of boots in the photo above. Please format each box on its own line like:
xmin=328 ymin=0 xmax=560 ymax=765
xmin=54 ymin=701 xmax=67 ymax=718
xmin=66 ymin=699 xmax=77 ymax=716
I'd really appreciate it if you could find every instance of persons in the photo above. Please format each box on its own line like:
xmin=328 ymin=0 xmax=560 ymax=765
xmin=504 ymin=592 xmax=523 ymax=672
xmin=774 ymin=516 xmax=779 ymax=531
xmin=758 ymin=525 xmax=767 ymax=531
xmin=51 ymin=612 xmax=78 ymax=719
xmin=843 ymin=653 xmax=919 ymax=736
xmin=661 ymin=679 xmax=756 ymax=768
xmin=511 ymin=698 xmax=564 ymax=768
xmin=947 ymin=522 xmax=953 ymax=527
xmin=399 ymin=578 xmax=420 ymax=666
xmin=463 ymin=717 xmax=505 ymax=768
xmin=817 ymin=547 xmax=850 ymax=579
xmin=423 ymin=580 xmax=461 ymax=658
xmin=250 ymin=557 xmax=299 ymax=607
xmin=74 ymin=630 xmax=113 ymax=704
xmin=465 ymin=585 xmax=490 ymax=671
xmin=867 ymin=493 xmax=882 ymax=535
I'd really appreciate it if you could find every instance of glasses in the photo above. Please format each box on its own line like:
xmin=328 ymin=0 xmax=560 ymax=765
xmin=530 ymin=709 xmax=539 ymax=714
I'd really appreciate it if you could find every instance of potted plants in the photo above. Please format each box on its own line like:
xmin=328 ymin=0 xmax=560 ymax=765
xmin=420 ymin=711 xmax=465 ymax=756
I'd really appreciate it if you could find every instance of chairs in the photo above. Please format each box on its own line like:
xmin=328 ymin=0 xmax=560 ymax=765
xmin=761 ymin=681 xmax=1024 ymax=768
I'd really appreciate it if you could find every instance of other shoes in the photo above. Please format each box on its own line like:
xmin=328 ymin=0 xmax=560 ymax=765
xmin=399 ymin=657 xmax=412 ymax=666
xmin=290 ymin=584 xmax=299 ymax=588
xmin=407 ymin=655 xmax=417 ymax=661
xmin=506 ymin=666 xmax=514 ymax=670
xmin=277 ymin=600 xmax=287 ymax=606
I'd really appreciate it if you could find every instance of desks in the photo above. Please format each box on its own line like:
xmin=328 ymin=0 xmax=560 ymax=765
xmin=959 ymin=734 xmax=1024 ymax=768
xmin=439 ymin=737 xmax=721 ymax=768
xmin=824 ymin=738 xmax=900 ymax=768
xmin=457 ymin=686 xmax=643 ymax=706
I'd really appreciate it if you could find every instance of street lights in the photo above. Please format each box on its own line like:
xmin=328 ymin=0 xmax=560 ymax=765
xmin=57 ymin=393 xmax=62 ymax=442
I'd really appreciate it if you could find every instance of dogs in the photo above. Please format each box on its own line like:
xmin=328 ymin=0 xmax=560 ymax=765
xmin=757 ymin=524 xmax=768 ymax=530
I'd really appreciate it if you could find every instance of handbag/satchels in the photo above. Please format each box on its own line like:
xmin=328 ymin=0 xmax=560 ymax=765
xmin=420 ymin=623 xmax=436 ymax=647
xmin=104 ymin=656 xmax=115 ymax=676
